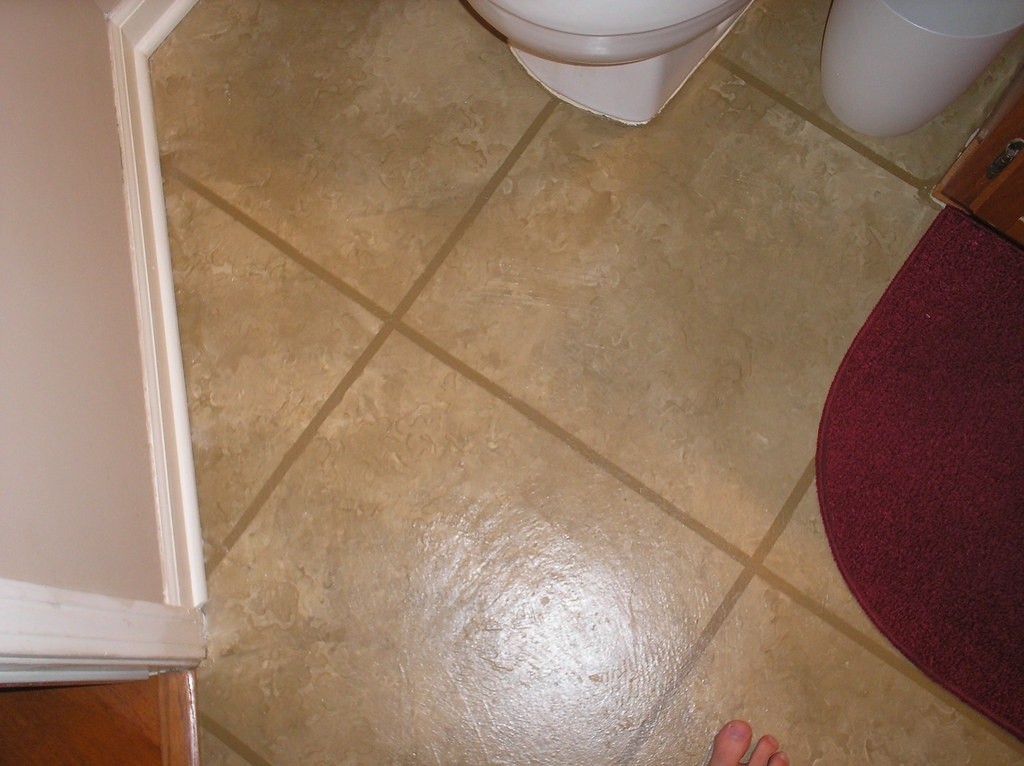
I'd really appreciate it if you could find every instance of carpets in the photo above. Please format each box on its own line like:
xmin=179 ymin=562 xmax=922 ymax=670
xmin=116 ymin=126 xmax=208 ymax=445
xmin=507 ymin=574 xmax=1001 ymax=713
xmin=815 ymin=205 xmax=1024 ymax=741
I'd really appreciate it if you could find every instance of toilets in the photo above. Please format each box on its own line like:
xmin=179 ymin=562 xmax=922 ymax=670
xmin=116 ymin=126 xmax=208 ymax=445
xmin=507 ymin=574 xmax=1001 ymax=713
xmin=465 ymin=0 xmax=756 ymax=126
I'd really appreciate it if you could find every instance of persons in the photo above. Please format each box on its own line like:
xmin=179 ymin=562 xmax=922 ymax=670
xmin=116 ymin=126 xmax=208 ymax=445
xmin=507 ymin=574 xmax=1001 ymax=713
xmin=708 ymin=720 xmax=789 ymax=766
